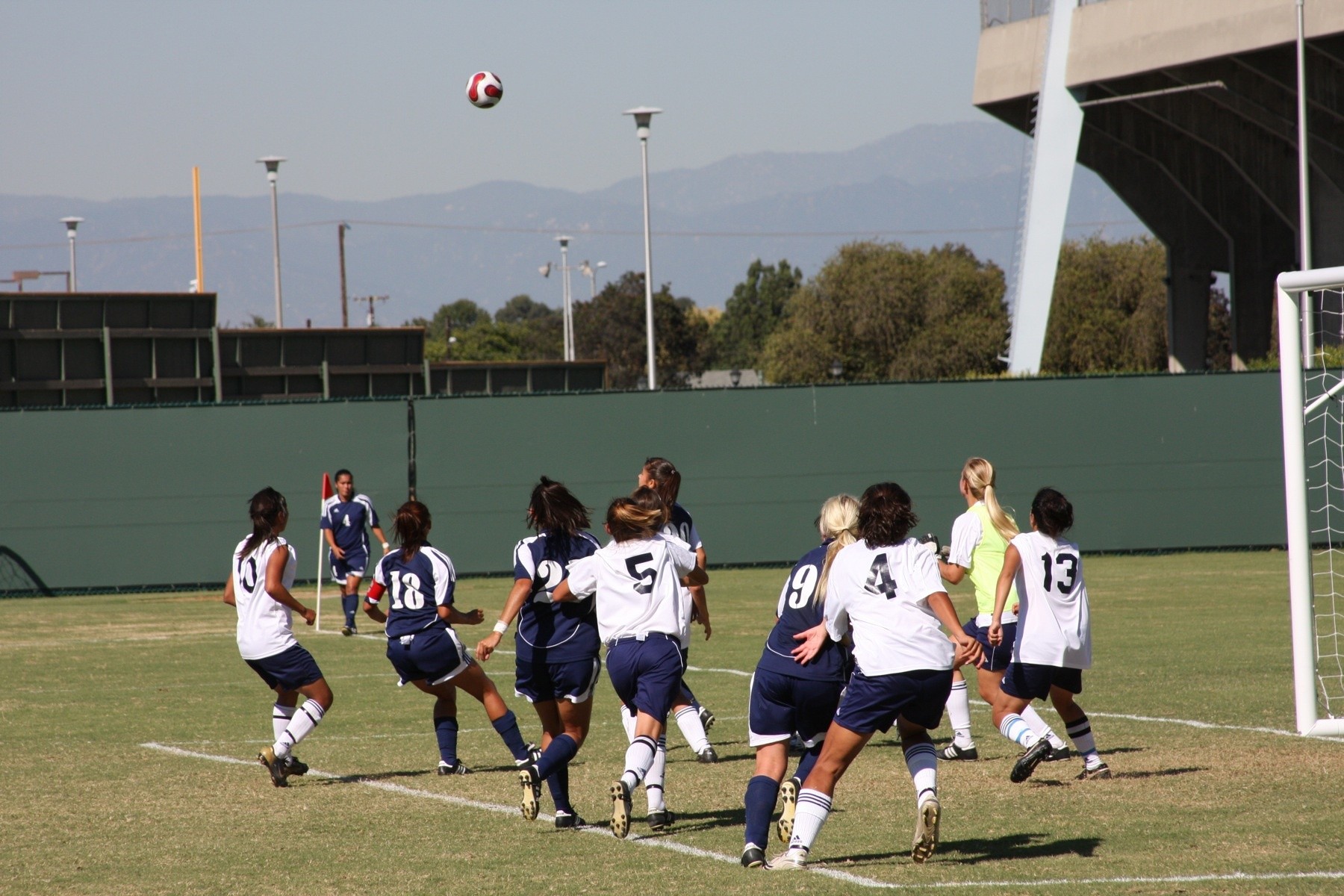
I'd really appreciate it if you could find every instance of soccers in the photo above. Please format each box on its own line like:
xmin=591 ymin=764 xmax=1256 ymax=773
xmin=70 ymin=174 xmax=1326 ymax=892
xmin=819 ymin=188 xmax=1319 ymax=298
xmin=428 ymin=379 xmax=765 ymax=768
xmin=465 ymin=71 xmax=503 ymax=109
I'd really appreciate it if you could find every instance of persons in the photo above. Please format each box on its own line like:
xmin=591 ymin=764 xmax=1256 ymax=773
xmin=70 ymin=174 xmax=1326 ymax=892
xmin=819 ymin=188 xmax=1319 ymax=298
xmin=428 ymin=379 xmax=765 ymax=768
xmin=320 ymin=469 xmax=392 ymax=638
xmin=225 ymin=487 xmax=333 ymax=788
xmin=741 ymin=481 xmax=989 ymax=873
xmin=919 ymin=456 xmax=1071 ymax=762
xmin=987 ymin=485 xmax=1111 ymax=784
xmin=552 ymin=458 xmax=719 ymax=840
xmin=475 ymin=475 xmax=604 ymax=829
xmin=363 ymin=501 xmax=542 ymax=776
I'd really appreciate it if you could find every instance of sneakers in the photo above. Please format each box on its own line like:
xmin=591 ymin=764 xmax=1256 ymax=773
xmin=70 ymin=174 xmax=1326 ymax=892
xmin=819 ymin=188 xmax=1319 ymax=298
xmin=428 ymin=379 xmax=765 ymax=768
xmin=555 ymin=804 xmax=586 ymax=828
xmin=1044 ymin=740 xmax=1071 ymax=762
xmin=284 ymin=756 xmax=308 ymax=775
xmin=777 ymin=776 xmax=802 ymax=842
xmin=257 ymin=745 xmax=289 ymax=787
xmin=437 ymin=758 xmax=474 ymax=778
xmin=1075 ymin=762 xmax=1110 ymax=780
xmin=761 ymin=848 xmax=808 ymax=872
xmin=350 ymin=624 xmax=358 ymax=633
xmin=341 ymin=626 xmax=352 ymax=635
xmin=647 ymin=807 xmax=675 ymax=831
xmin=741 ymin=847 xmax=764 ymax=868
xmin=1010 ymin=737 xmax=1053 ymax=783
xmin=696 ymin=748 xmax=718 ymax=763
xmin=698 ymin=709 xmax=715 ymax=736
xmin=609 ymin=780 xmax=633 ymax=838
xmin=518 ymin=766 xmax=542 ymax=821
xmin=935 ymin=741 xmax=978 ymax=762
xmin=515 ymin=742 xmax=542 ymax=771
xmin=912 ymin=793 xmax=941 ymax=864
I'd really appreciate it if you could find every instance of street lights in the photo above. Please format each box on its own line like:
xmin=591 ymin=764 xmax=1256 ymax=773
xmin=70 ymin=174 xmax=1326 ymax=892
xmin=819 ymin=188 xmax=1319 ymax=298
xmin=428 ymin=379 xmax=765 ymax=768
xmin=577 ymin=260 xmax=609 ymax=300
xmin=555 ymin=235 xmax=572 ymax=362
xmin=255 ymin=154 xmax=286 ymax=330
xmin=623 ymin=104 xmax=661 ymax=390
xmin=60 ymin=216 xmax=86 ymax=293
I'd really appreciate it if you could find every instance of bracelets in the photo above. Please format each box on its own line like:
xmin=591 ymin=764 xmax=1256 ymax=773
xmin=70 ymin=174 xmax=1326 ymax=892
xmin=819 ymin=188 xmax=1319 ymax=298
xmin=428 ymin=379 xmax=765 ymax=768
xmin=493 ymin=621 xmax=507 ymax=635
xmin=381 ymin=541 xmax=390 ymax=549
